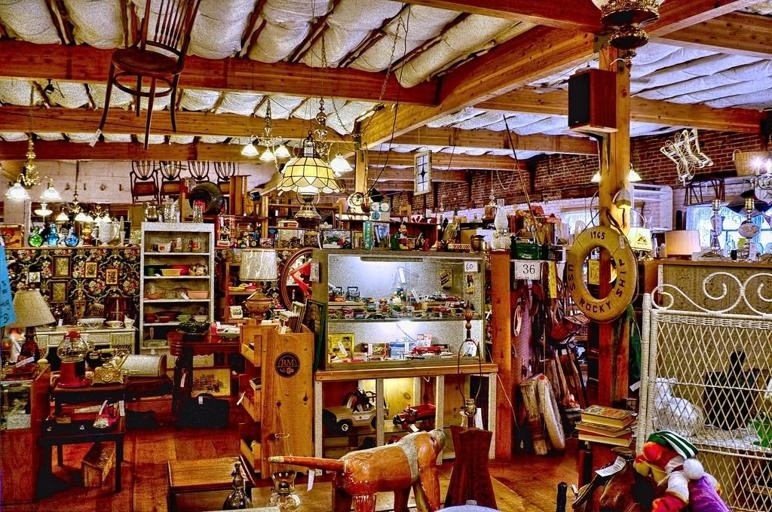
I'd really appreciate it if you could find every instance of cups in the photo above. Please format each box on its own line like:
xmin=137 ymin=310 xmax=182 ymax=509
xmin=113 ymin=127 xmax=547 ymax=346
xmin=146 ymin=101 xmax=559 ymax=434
xmin=124 ymin=319 xmax=135 ymax=330
xmin=105 ymin=320 xmax=124 ymax=329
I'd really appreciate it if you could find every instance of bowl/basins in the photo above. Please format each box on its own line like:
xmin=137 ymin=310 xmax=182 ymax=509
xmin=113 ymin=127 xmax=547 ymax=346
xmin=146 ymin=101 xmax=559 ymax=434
xmin=188 ymin=290 xmax=209 ymax=299
xmin=78 ymin=318 xmax=107 ymax=330
xmin=160 ymin=268 xmax=185 ymax=276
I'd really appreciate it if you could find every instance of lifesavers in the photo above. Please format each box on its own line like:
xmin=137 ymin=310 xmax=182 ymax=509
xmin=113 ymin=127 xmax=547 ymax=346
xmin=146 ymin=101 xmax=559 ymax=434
xmin=538 ymin=376 xmax=565 ymax=449
xmin=567 ymin=227 xmax=636 ymax=320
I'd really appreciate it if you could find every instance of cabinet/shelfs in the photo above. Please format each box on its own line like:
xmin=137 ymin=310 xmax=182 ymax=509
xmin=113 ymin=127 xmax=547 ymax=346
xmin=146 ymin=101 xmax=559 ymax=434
xmin=219 ymin=196 xmax=498 ymax=480
xmin=137 ymin=221 xmax=214 ymax=356
xmin=35 ymin=371 xmax=128 ymax=492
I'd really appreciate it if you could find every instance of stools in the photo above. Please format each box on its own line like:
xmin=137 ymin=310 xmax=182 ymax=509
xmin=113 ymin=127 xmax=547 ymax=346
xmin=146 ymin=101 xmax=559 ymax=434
xmin=163 ymin=455 xmax=255 ymax=510
xmin=122 ymin=373 xmax=172 ymax=426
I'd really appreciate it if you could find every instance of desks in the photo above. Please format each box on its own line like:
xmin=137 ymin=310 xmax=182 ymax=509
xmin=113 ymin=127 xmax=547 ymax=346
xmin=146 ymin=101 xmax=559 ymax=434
xmin=0 ymin=362 xmax=51 ymax=504
xmin=162 ymin=331 xmax=240 ymax=423
xmin=22 ymin=323 xmax=133 ymax=360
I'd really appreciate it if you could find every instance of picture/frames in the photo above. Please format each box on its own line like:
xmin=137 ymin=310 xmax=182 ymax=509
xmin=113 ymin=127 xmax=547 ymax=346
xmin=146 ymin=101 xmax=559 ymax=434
xmin=48 ymin=255 xmax=73 ymax=304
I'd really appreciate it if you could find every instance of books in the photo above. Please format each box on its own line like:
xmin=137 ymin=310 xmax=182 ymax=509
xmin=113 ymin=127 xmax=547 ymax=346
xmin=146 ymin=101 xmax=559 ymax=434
xmin=577 ymin=422 xmax=633 ymax=438
xmin=579 ymin=404 xmax=635 ymax=428
xmin=574 ymin=431 xmax=635 ymax=447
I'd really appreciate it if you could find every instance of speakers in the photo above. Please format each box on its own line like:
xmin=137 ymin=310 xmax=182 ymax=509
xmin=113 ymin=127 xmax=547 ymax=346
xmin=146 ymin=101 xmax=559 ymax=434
xmin=567 ymin=68 xmax=616 ymax=133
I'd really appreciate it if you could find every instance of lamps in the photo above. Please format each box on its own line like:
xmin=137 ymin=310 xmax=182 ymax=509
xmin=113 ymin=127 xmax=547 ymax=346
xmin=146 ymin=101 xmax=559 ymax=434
xmin=2 ymin=77 xmax=94 ymax=224
xmin=7 ymin=289 xmax=55 ymax=364
xmin=240 ymin=29 xmax=353 ymax=232
xmin=626 ymin=227 xmax=653 ymax=261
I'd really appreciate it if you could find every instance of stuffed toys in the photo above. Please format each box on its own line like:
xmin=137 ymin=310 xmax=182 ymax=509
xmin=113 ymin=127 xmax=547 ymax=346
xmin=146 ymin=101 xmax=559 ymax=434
xmin=631 ymin=428 xmax=732 ymax=511
